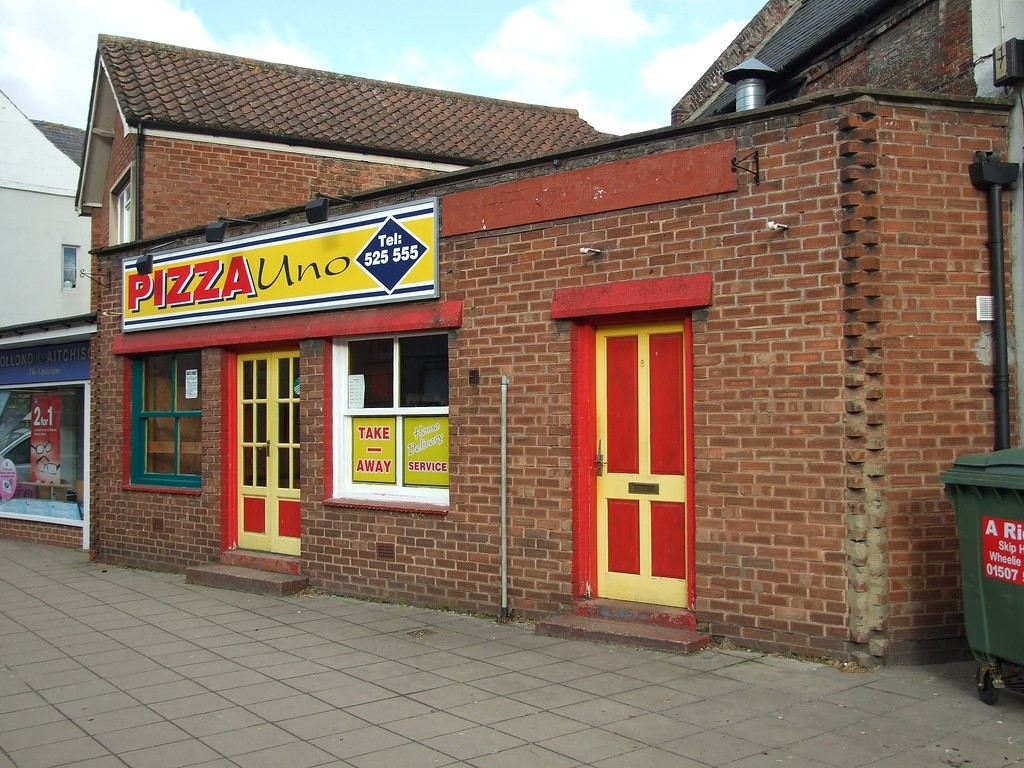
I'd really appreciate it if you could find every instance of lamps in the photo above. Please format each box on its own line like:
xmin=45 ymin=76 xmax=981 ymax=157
xmin=306 ymin=193 xmax=360 ymax=224
xmin=136 ymin=238 xmax=183 ymax=276
xmin=206 ymin=216 xmax=260 ymax=242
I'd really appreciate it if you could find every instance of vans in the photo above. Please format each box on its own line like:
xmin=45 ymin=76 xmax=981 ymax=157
xmin=0 ymin=428 xmax=77 ymax=501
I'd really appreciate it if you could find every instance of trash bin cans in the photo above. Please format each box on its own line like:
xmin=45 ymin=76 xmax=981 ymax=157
xmin=937 ymin=445 xmax=1024 ymax=705
